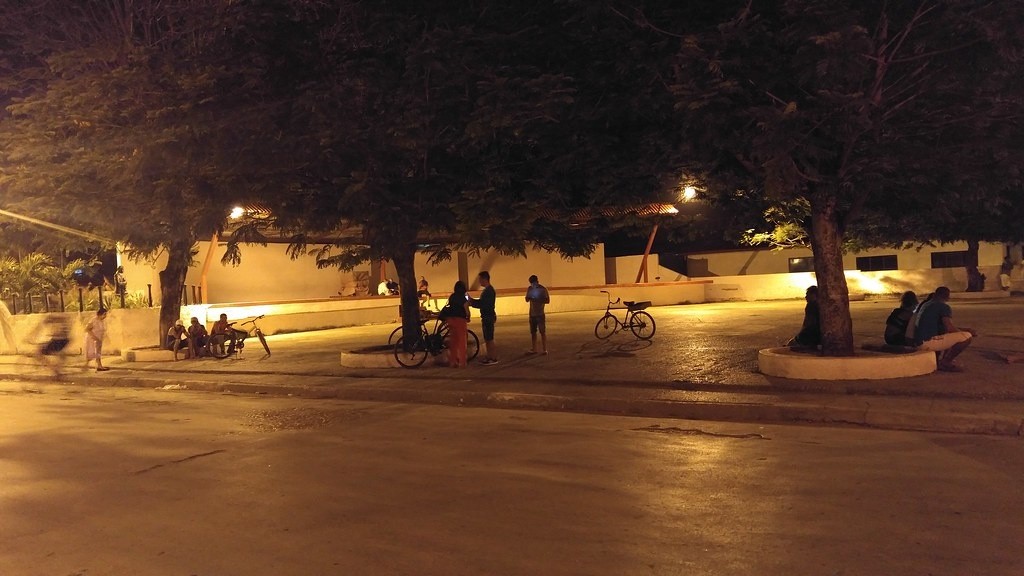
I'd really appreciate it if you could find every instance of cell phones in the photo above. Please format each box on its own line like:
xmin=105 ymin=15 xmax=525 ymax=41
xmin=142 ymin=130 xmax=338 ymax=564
xmin=465 ymin=296 xmax=469 ymax=301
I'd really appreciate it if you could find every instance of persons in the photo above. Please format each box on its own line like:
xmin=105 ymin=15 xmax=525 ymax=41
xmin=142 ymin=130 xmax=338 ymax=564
xmin=417 ymin=276 xmax=431 ymax=307
xmin=525 ymin=274 xmax=551 ymax=354
xmin=446 ymin=280 xmax=473 ymax=367
xmin=165 ymin=320 xmax=194 ymax=362
xmin=378 ymin=278 xmax=400 ymax=296
xmin=884 ymin=286 xmax=973 ymax=373
xmin=999 ymin=256 xmax=1014 ymax=291
xmin=188 ymin=316 xmax=212 ymax=359
xmin=468 ymin=270 xmax=498 ymax=365
xmin=210 ymin=313 xmax=238 ymax=354
xmin=787 ymin=286 xmax=822 ymax=345
xmin=82 ymin=307 xmax=111 ymax=371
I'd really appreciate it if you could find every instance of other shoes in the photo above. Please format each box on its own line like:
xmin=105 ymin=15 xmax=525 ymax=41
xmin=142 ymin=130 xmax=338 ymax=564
xmin=455 ymin=361 xmax=458 ymax=365
xmin=227 ymin=348 xmax=238 ymax=353
xmin=459 ymin=362 xmax=467 ymax=368
xmin=221 ymin=350 xmax=225 ymax=354
xmin=97 ymin=367 xmax=109 ymax=371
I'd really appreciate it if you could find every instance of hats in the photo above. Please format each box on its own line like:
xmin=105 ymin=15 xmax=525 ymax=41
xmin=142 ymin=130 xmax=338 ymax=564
xmin=191 ymin=317 xmax=198 ymax=324
xmin=176 ymin=319 xmax=183 ymax=326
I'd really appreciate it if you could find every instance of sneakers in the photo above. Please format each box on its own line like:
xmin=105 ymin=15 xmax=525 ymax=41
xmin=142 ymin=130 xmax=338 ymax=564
xmin=528 ymin=350 xmax=538 ymax=353
xmin=940 ymin=358 xmax=955 ymax=364
xmin=543 ymin=349 xmax=547 ymax=354
xmin=477 ymin=355 xmax=498 ymax=365
xmin=940 ymin=364 xmax=961 ymax=372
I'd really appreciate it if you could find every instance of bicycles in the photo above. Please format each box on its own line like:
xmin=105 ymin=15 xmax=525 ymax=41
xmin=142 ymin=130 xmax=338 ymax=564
xmin=388 ymin=312 xmax=481 ymax=368
xmin=206 ymin=314 xmax=271 ymax=359
xmin=594 ymin=289 xmax=657 ymax=340
